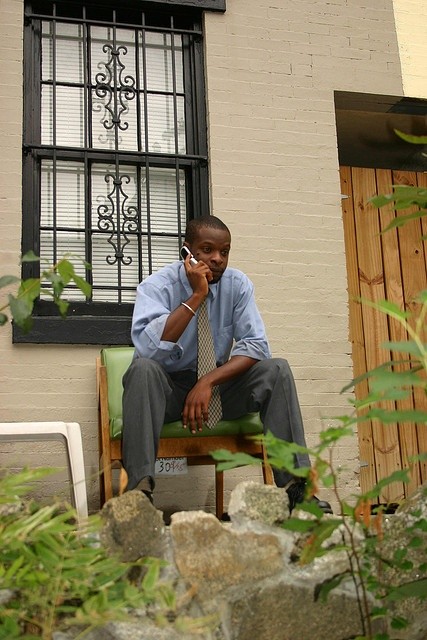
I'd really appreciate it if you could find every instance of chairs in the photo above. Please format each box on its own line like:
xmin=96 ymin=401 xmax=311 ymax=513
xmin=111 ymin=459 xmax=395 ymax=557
xmin=94 ymin=345 xmax=274 ymax=522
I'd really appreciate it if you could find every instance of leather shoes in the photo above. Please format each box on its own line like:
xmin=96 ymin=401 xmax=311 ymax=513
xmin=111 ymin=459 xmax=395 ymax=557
xmin=285 ymin=476 xmax=334 ymax=514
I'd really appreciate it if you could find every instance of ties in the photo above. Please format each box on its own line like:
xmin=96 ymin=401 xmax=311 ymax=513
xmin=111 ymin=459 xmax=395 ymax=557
xmin=197 ymin=294 xmax=224 ymax=431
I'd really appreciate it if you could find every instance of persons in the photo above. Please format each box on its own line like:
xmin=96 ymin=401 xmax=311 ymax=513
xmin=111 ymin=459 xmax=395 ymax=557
xmin=120 ymin=215 xmax=333 ymax=514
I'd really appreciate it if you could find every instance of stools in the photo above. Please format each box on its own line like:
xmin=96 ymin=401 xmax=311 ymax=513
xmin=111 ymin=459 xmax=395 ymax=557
xmin=0 ymin=422 xmax=89 ymax=521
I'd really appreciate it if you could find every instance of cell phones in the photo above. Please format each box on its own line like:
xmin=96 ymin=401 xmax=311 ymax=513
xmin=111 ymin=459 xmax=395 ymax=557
xmin=179 ymin=246 xmax=198 ymax=267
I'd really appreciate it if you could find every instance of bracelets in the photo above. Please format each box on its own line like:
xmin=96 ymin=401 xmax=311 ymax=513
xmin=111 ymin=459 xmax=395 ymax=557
xmin=181 ymin=302 xmax=196 ymax=316
